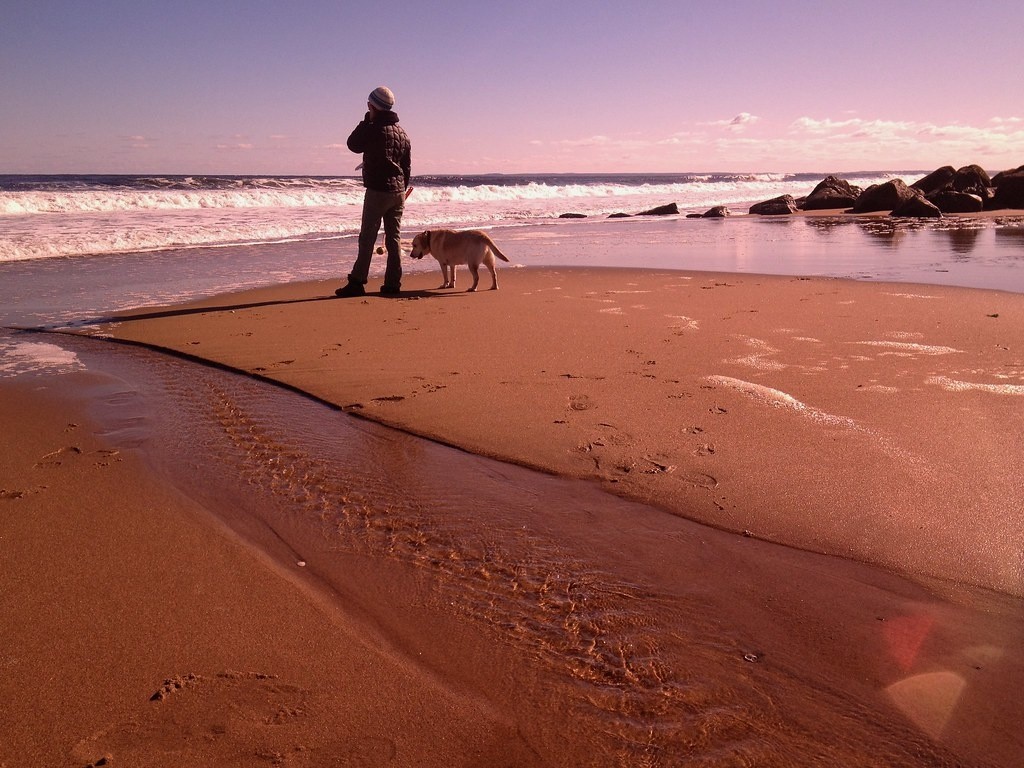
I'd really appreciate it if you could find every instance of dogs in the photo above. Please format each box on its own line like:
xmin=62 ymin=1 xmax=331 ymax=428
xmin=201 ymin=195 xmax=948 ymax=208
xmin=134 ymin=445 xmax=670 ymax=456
xmin=410 ymin=228 xmax=510 ymax=292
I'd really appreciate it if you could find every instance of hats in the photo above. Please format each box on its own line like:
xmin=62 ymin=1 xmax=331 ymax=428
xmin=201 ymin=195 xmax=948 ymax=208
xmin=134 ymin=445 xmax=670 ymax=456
xmin=368 ymin=86 xmax=394 ymax=110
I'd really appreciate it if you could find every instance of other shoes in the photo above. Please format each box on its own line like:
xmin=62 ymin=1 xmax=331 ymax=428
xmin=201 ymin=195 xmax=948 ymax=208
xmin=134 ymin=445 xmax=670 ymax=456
xmin=380 ymin=286 xmax=400 ymax=296
xmin=335 ymin=282 xmax=366 ymax=297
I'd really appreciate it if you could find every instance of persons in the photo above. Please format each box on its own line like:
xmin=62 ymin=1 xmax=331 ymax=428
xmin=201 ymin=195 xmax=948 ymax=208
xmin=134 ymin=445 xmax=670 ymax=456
xmin=335 ymin=87 xmax=412 ymax=296
